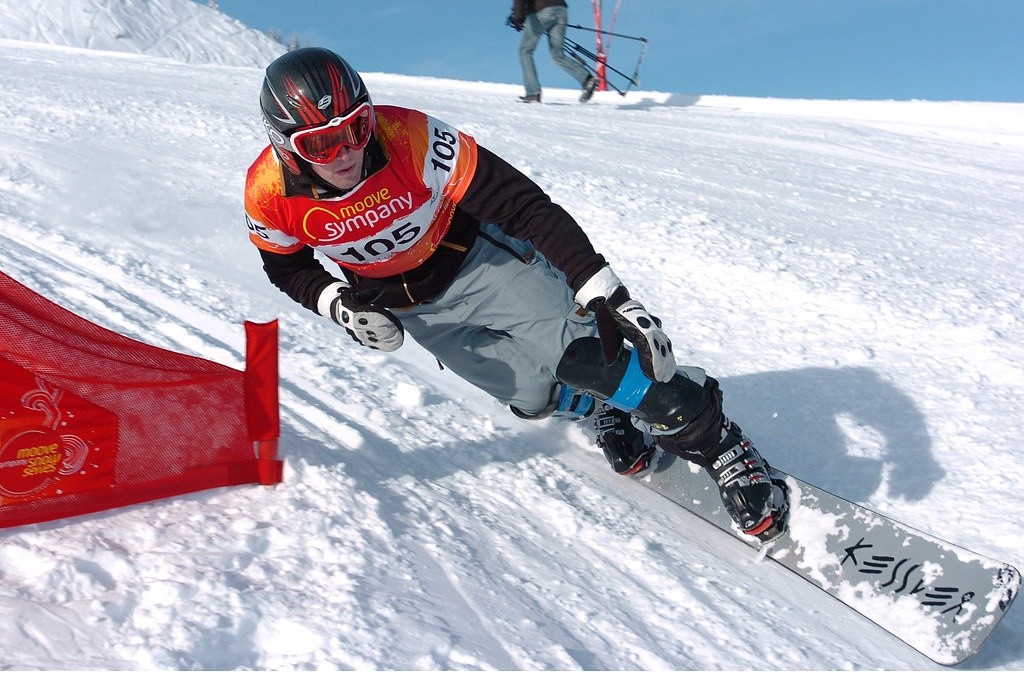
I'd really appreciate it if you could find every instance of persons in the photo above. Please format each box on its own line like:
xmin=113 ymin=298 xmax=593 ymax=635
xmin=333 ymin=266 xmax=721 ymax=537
xmin=505 ymin=0 xmax=600 ymax=103
xmin=244 ymin=47 xmax=786 ymax=543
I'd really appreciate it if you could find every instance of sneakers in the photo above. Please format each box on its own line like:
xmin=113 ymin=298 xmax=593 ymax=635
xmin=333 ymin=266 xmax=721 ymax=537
xmin=571 ymin=397 xmax=645 ymax=476
xmin=579 ymin=76 xmax=600 ymax=103
xmin=518 ymin=93 xmax=540 ymax=103
xmin=685 ymin=413 xmax=773 ymax=531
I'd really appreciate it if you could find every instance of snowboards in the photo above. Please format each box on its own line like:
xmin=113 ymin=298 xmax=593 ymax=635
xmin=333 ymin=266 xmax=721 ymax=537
xmin=559 ymin=397 xmax=1022 ymax=666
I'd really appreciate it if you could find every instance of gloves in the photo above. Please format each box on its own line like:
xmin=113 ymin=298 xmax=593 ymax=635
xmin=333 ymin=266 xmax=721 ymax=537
xmin=572 ymin=261 xmax=676 ymax=384
xmin=316 ymin=280 xmax=404 ymax=352
xmin=508 ymin=16 xmax=522 ymax=31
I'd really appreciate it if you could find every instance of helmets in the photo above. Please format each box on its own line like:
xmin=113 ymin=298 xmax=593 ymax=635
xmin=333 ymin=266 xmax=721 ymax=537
xmin=259 ymin=47 xmax=377 ymax=177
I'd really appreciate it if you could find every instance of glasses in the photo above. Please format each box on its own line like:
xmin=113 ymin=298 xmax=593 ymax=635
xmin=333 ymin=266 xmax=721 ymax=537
xmin=290 ymin=101 xmax=375 ymax=166
